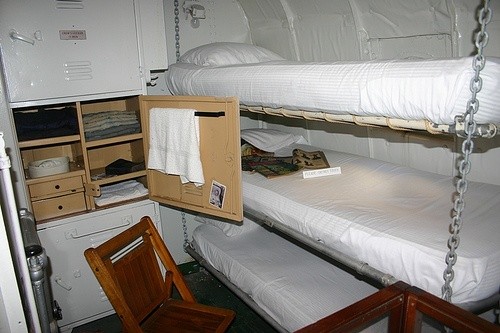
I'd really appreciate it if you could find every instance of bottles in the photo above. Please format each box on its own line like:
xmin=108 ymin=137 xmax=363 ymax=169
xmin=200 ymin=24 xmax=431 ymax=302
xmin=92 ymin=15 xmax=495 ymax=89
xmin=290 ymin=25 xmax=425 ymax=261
xmin=18 ymin=209 xmax=43 ymax=259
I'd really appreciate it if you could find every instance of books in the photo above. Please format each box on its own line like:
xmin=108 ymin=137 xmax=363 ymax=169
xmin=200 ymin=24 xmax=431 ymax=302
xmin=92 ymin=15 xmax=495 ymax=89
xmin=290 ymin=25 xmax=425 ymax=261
xmin=239 ymin=148 xmax=330 ymax=179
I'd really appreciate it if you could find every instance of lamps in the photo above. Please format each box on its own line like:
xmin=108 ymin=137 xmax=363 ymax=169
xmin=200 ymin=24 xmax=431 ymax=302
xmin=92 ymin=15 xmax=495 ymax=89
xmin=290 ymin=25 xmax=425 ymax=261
xmin=190 ymin=5 xmax=207 ymax=28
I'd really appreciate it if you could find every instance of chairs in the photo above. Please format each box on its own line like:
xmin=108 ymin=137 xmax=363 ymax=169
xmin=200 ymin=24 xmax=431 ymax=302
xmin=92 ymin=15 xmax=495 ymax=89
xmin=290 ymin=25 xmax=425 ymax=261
xmin=83 ymin=215 xmax=237 ymax=333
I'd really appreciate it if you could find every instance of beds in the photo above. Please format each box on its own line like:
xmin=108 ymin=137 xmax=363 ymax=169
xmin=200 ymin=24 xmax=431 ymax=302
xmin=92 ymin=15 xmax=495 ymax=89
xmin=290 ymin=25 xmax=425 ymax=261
xmin=164 ymin=61 xmax=500 ymax=140
xmin=189 ymin=226 xmax=496 ymax=333
xmin=240 ymin=143 xmax=500 ymax=313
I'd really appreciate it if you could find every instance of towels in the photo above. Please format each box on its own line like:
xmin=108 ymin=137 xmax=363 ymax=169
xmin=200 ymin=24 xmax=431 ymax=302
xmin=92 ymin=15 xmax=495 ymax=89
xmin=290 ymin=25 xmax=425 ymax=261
xmin=146 ymin=108 xmax=205 ymax=187
xmin=94 ymin=179 xmax=149 ymax=207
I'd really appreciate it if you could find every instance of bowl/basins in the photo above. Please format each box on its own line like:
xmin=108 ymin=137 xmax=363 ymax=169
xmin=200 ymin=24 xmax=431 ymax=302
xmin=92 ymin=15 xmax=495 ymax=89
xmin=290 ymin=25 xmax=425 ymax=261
xmin=27 ymin=157 xmax=70 ymax=178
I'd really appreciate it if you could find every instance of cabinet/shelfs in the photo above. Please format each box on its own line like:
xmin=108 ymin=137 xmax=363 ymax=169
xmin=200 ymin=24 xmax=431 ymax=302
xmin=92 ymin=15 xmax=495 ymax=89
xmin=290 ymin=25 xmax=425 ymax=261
xmin=36 ymin=203 xmax=166 ymax=327
xmin=0 ymin=0 xmax=146 ymax=105
xmin=9 ymin=95 xmax=245 ymax=225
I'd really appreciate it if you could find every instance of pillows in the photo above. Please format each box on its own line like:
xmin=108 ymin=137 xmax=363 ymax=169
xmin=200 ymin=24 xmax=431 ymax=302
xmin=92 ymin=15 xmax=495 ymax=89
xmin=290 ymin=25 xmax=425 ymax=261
xmin=195 ymin=210 xmax=262 ymax=237
xmin=180 ymin=42 xmax=283 ymax=67
